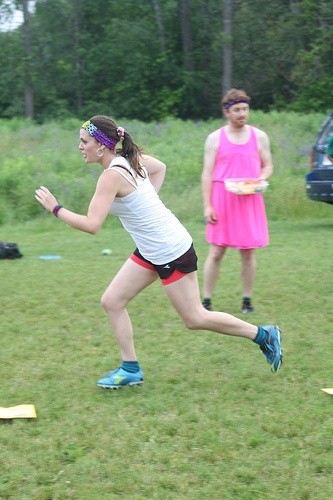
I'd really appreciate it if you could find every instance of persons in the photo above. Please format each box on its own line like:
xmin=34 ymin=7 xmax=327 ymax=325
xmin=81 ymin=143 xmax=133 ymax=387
xmin=35 ymin=113 xmax=282 ymax=388
xmin=200 ymin=89 xmax=272 ymax=313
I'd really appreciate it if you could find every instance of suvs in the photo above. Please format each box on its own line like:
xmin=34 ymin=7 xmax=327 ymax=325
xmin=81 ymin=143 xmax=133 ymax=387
xmin=305 ymin=111 xmax=333 ymax=206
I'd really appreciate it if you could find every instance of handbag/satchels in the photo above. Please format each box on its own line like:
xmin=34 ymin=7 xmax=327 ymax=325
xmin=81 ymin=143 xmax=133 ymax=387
xmin=0 ymin=242 xmax=23 ymax=260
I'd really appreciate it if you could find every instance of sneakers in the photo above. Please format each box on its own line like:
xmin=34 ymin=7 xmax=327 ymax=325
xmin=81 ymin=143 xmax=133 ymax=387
xmin=260 ymin=325 xmax=283 ymax=375
xmin=96 ymin=368 xmax=144 ymax=390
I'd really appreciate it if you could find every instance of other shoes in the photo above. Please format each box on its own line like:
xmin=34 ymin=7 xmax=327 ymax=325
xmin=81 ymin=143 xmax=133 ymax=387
xmin=241 ymin=306 xmax=254 ymax=314
xmin=203 ymin=301 xmax=211 ymax=310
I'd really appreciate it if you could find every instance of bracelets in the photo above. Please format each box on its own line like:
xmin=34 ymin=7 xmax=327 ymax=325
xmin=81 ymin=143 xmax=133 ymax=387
xmin=53 ymin=205 xmax=62 ymax=217
